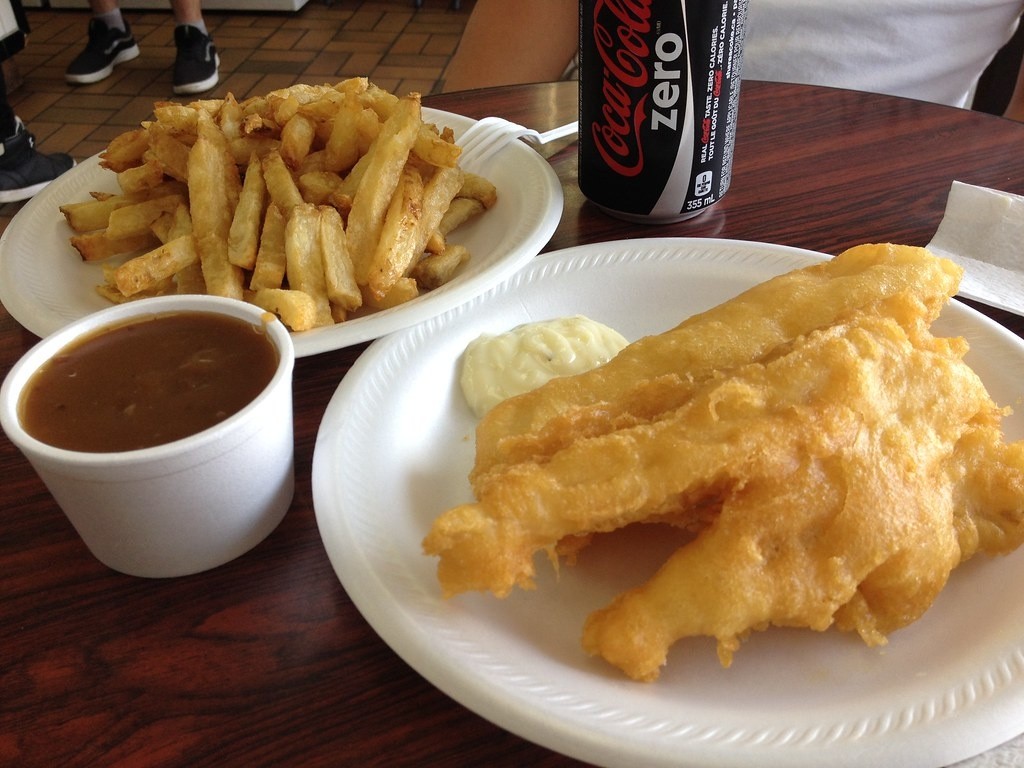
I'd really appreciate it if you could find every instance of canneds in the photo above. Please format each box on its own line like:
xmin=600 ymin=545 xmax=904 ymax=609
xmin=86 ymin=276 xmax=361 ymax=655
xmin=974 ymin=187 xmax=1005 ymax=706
xmin=572 ymin=0 xmax=749 ymax=223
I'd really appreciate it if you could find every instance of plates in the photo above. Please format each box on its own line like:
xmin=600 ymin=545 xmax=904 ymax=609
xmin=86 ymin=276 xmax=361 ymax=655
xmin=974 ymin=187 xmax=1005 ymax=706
xmin=312 ymin=236 xmax=1024 ymax=768
xmin=0 ymin=106 xmax=565 ymax=359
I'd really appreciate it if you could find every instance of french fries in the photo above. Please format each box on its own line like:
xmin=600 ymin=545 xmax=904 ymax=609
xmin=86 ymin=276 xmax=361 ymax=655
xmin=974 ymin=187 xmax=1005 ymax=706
xmin=58 ymin=72 xmax=497 ymax=330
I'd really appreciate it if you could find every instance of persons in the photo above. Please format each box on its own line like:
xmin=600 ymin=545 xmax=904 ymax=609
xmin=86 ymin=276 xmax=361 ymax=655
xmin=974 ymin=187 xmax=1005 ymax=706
xmin=62 ymin=0 xmax=222 ymax=94
xmin=443 ymin=0 xmax=1024 ymax=123
xmin=0 ymin=0 xmax=77 ymax=204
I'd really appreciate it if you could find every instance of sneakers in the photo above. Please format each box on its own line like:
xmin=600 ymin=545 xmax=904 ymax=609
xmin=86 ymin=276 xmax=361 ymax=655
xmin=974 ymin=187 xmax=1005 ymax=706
xmin=171 ymin=24 xmax=220 ymax=95
xmin=0 ymin=114 xmax=78 ymax=205
xmin=64 ymin=17 xmax=141 ymax=85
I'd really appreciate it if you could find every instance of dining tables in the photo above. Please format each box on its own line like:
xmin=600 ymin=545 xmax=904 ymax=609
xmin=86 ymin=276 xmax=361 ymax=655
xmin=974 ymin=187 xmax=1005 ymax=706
xmin=0 ymin=78 xmax=1024 ymax=766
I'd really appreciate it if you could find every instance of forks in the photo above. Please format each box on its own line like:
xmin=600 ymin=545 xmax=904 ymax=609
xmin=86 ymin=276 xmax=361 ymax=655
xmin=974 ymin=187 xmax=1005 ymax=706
xmin=453 ymin=117 xmax=579 ymax=171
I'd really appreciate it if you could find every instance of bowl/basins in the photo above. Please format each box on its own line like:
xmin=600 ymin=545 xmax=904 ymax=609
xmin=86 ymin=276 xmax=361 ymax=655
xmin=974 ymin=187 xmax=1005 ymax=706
xmin=1 ymin=294 xmax=296 ymax=579
